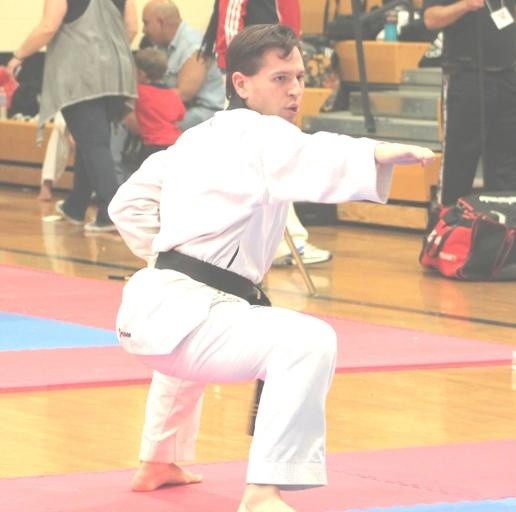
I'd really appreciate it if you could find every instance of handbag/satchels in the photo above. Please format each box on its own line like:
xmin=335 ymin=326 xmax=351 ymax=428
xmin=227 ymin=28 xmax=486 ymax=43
xmin=418 ymin=185 xmax=516 ymax=281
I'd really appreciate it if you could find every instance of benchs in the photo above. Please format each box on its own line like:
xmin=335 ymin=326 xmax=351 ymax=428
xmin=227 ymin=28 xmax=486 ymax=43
xmin=2 ymin=31 xmax=431 ymax=191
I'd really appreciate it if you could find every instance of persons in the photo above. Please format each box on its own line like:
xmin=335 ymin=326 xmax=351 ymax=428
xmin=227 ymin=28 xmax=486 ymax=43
xmin=108 ymin=25 xmax=441 ymax=512
xmin=320 ymin=0 xmax=516 ymax=204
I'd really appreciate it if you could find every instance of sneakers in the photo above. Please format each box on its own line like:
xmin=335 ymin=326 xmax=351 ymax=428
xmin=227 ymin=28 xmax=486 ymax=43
xmin=55 ymin=200 xmax=117 ymax=232
xmin=271 ymin=241 xmax=331 ymax=266
